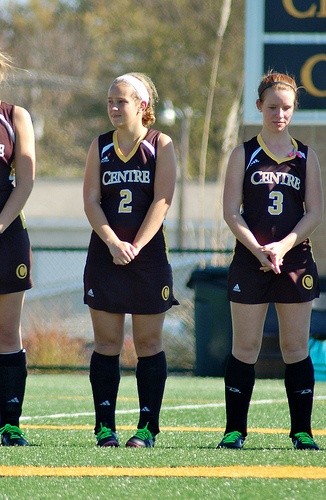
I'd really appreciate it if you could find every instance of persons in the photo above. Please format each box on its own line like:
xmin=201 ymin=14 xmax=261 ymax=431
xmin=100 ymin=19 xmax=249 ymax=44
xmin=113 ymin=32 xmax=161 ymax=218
xmin=81 ymin=73 xmax=180 ymax=448
xmin=0 ymin=50 xmax=35 ymax=447
xmin=216 ymin=73 xmax=321 ymax=450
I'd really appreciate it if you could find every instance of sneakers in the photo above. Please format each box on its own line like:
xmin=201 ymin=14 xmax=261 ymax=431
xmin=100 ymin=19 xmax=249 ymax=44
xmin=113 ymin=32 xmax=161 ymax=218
xmin=292 ymin=432 xmax=319 ymax=450
xmin=0 ymin=424 xmax=28 ymax=446
xmin=95 ymin=422 xmax=120 ymax=447
xmin=216 ymin=431 xmax=245 ymax=449
xmin=126 ymin=422 xmax=155 ymax=448
